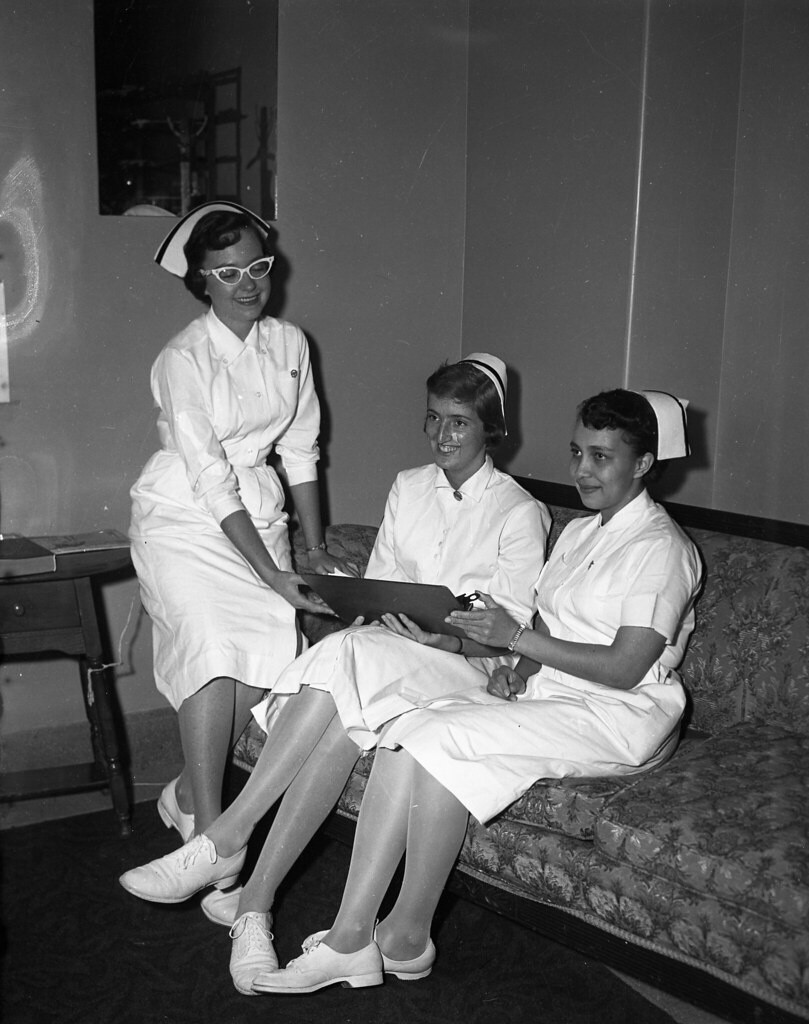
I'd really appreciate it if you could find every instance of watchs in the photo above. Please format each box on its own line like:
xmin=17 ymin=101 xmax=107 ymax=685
xmin=306 ymin=542 xmax=327 ymax=552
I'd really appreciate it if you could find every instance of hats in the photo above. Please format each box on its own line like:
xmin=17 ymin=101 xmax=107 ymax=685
xmin=627 ymin=389 xmax=692 ymax=461
xmin=153 ymin=200 xmax=271 ymax=278
xmin=457 ymin=352 xmax=508 ymax=436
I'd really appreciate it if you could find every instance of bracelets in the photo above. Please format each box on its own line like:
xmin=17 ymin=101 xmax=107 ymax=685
xmin=454 ymin=635 xmax=465 ymax=654
xmin=507 ymin=623 xmax=529 ymax=652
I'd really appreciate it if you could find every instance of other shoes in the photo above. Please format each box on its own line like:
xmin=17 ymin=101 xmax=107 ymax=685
xmin=300 ymin=918 xmax=437 ymax=980
xmin=228 ymin=911 xmax=279 ymax=995
xmin=199 ymin=881 xmax=273 ymax=927
xmin=157 ymin=776 xmax=240 ymax=889
xmin=118 ymin=833 xmax=248 ymax=903
xmin=250 ymin=938 xmax=383 ymax=993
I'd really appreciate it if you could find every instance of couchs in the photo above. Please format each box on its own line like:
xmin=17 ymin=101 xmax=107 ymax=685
xmin=225 ymin=481 xmax=809 ymax=1024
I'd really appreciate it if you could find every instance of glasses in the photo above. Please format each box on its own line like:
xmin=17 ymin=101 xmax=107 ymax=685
xmin=198 ymin=256 xmax=275 ymax=286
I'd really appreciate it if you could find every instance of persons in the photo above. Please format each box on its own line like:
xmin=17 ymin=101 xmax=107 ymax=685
xmin=127 ymin=201 xmax=360 ymax=928
xmin=251 ymin=387 xmax=701 ymax=993
xmin=118 ymin=352 xmax=551 ymax=995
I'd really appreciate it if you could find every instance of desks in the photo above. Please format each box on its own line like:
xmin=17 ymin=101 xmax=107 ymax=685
xmin=0 ymin=528 xmax=135 ymax=854
